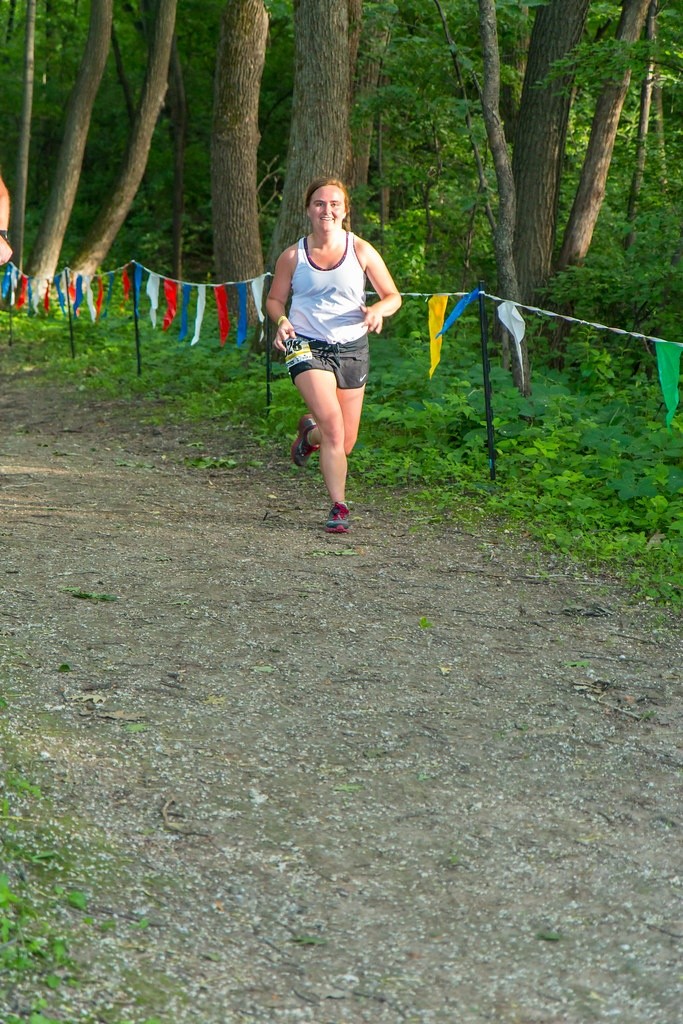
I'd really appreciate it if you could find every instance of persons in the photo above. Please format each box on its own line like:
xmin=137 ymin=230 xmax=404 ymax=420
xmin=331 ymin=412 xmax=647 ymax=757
xmin=0 ymin=175 xmax=13 ymax=266
xmin=265 ymin=178 xmax=402 ymax=533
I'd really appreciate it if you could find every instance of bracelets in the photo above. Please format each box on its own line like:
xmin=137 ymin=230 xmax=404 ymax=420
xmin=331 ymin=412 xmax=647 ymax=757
xmin=277 ymin=316 xmax=288 ymax=326
xmin=0 ymin=230 xmax=10 ymax=245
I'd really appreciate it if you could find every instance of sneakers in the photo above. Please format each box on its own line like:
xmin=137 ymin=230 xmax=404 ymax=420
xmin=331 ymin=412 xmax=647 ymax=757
xmin=325 ymin=502 xmax=349 ymax=533
xmin=292 ymin=413 xmax=320 ymax=467
